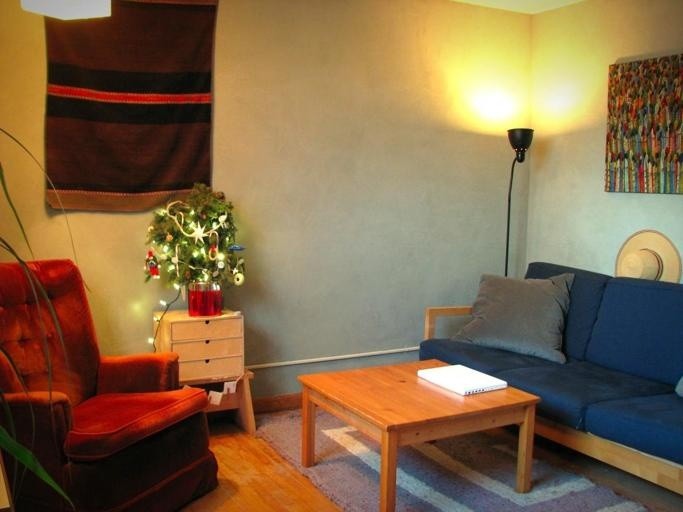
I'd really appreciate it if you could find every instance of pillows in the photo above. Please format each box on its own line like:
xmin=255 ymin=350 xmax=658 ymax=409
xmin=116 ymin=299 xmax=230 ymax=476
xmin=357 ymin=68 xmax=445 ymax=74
xmin=451 ymin=270 xmax=575 ymax=369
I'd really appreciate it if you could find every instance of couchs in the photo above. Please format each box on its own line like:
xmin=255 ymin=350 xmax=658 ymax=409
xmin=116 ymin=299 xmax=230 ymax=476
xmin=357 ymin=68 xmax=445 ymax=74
xmin=422 ymin=259 xmax=681 ymax=498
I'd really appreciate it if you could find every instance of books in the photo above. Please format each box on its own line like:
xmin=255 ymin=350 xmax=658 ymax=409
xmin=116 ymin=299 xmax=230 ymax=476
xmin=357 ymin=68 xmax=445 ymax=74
xmin=417 ymin=376 xmax=507 ymax=403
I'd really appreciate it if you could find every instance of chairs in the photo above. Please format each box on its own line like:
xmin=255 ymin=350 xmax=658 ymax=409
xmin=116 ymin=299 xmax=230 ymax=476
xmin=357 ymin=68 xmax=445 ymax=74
xmin=0 ymin=257 xmax=220 ymax=512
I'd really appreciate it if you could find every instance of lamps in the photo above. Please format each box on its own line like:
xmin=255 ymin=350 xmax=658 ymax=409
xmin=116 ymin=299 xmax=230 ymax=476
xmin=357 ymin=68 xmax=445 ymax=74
xmin=503 ymin=127 xmax=534 ymax=276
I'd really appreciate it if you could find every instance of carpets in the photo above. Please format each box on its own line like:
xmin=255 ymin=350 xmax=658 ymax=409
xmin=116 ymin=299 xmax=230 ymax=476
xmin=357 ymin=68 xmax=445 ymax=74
xmin=254 ymin=405 xmax=649 ymax=512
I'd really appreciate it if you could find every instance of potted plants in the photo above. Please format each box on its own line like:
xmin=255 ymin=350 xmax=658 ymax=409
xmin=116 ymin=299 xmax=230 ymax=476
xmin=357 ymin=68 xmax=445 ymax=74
xmin=141 ymin=180 xmax=247 ymax=317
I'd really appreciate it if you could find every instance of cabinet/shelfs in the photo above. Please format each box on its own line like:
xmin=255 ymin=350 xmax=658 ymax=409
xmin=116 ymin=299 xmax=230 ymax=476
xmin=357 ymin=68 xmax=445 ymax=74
xmin=151 ymin=308 xmax=245 ymax=381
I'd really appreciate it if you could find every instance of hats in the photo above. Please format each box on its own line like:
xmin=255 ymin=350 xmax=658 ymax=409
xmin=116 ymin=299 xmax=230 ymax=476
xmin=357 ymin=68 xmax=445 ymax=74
xmin=615 ymin=229 xmax=682 ymax=284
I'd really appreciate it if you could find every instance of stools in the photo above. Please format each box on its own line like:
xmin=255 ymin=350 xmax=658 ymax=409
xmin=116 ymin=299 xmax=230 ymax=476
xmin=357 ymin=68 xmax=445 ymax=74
xmin=177 ymin=367 xmax=256 ymax=441
